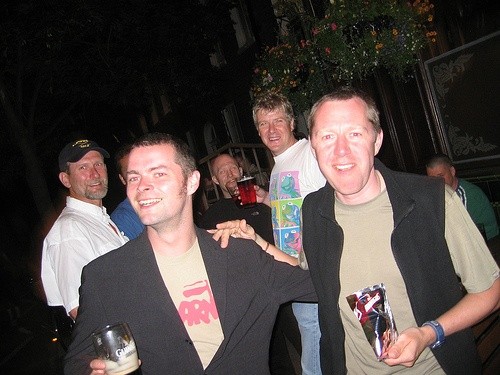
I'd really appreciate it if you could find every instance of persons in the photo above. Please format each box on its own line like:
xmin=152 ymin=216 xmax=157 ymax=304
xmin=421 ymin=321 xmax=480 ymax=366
xmin=63 ymin=135 xmax=319 ymax=375
xmin=31 ymin=89 xmax=500 ymax=375
xmin=206 ymin=88 xmax=500 ymax=375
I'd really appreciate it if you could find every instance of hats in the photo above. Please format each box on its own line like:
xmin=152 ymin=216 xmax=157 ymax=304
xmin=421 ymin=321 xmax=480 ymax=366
xmin=58 ymin=139 xmax=110 ymax=168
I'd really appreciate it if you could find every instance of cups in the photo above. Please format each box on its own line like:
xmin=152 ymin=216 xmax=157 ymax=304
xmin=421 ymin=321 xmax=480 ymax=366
xmin=225 ymin=179 xmax=244 ymax=209
xmin=91 ymin=321 xmax=139 ymax=375
xmin=235 ymin=176 xmax=257 ymax=209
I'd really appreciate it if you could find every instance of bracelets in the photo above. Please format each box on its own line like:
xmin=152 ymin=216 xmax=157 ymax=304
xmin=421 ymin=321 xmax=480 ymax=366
xmin=263 ymin=241 xmax=270 ymax=252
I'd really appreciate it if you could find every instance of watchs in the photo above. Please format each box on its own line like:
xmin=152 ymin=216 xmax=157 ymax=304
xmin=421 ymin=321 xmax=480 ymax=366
xmin=421 ymin=319 xmax=447 ymax=350
